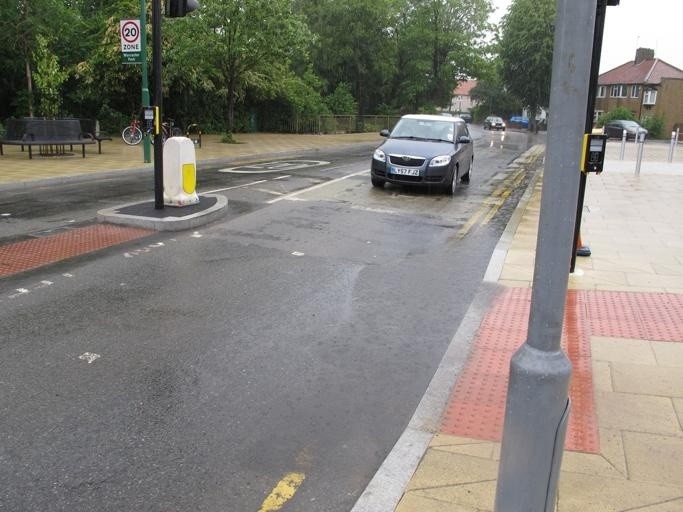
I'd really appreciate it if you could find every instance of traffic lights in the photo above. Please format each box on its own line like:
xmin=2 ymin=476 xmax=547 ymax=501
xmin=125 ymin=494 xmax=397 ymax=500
xmin=164 ymin=0 xmax=199 ymax=17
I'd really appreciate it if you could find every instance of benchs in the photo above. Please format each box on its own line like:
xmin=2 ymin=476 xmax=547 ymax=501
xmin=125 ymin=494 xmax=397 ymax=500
xmin=21 ymin=119 xmax=85 ymax=158
xmin=0 ymin=119 xmax=21 ymax=156
xmin=62 ymin=117 xmax=113 ymax=155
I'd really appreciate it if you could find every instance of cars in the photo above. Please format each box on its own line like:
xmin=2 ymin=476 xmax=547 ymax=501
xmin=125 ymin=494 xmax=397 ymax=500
xmin=440 ymin=113 xmax=452 ymax=117
xmin=482 ymin=114 xmax=505 ymax=131
xmin=148 ymin=113 xmax=182 ymax=146
xmin=453 ymin=112 xmax=472 ymax=124
xmin=121 ymin=111 xmax=167 ymax=146
xmin=371 ymin=114 xmax=474 ymax=195
xmin=510 ymin=117 xmax=529 ymax=129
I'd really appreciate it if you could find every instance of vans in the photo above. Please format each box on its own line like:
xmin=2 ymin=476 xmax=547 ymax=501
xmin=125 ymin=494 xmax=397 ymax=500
xmin=603 ymin=120 xmax=648 ymax=140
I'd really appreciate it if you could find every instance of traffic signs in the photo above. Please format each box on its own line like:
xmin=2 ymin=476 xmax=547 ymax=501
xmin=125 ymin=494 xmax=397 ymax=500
xmin=118 ymin=18 xmax=140 ymax=52
